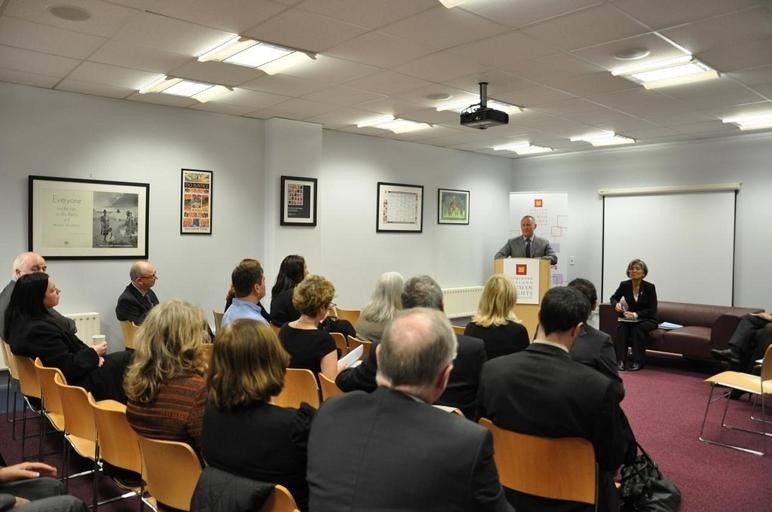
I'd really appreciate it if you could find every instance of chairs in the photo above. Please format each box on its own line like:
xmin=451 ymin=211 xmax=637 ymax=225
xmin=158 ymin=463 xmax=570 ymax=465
xmin=699 ymin=342 xmax=772 ymax=457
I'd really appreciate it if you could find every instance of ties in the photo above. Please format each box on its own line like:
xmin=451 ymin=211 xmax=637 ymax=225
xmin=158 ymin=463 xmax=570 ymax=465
xmin=525 ymin=238 xmax=531 ymax=257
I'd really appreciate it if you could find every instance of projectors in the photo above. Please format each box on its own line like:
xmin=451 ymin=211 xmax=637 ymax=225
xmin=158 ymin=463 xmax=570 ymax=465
xmin=461 ymin=109 xmax=508 ymax=129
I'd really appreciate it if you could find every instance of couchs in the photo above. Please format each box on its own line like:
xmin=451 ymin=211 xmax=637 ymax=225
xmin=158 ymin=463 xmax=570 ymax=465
xmin=599 ymin=301 xmax=772 ymax=369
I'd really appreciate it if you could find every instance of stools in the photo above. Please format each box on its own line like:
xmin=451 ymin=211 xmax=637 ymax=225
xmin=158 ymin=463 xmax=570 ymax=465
xmin=750 ymin=363 xmax=772 ymax=426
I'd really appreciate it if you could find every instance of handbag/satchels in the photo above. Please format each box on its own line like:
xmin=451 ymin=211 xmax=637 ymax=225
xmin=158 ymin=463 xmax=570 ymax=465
xmin=620 ymin=442 xmax=681 ymax=512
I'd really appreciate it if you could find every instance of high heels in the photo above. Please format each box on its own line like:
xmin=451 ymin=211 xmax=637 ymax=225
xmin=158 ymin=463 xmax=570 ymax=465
xmin=723 ymin=389 xmax=751 ymax=401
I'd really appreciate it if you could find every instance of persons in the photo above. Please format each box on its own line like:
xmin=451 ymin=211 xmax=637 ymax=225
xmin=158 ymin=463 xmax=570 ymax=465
xmin=100 ymin=206 xmax=136 ymax=244
xmin=201 ymin=323 xmax=316 ymax=511
xmin=562 ymin=276 xmax=625 ymax=406
xmin=0 ymin=462 xmax=92 ymax=511
xmin=221 ymin=255 xmax=530 ymax=423
xmin=119 ymin=298 xmax=214 ymax=449
xmin=7 ymin=271 xmax=133 ymax=400
xmin=607 ymin=258 xmax=658 ymax=372
xmin=492 ymin=215 xmax=558 ymax=266
xmin=115 ymin=261 xmax=160 ymax=326
xmin=303 ymin=305 xmax=512 ymax=509
xmin=2 ymin=253 xmax=50 ymax=344
xmin=709 ymin=307 xmax=770 ymax=402
xmin=473 ymin=288 xmax=636 ymax=511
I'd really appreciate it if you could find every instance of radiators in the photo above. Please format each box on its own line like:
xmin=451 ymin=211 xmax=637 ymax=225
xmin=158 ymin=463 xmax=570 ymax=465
xmin=440 ymin=285 xmax=484 ymax=321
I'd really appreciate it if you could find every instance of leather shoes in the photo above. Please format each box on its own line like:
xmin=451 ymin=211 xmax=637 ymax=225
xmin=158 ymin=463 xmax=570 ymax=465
xmin=628 ymin=361 xmax=643 ymax=371
xmin=711 ymin=348 xmax=740 ymax=366
xmin=618 ymin=360 xmax=626 ymax=371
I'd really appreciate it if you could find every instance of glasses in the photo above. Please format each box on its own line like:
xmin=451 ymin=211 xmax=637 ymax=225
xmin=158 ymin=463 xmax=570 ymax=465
xmin=136 ymin=272 xmax=156 ymax=279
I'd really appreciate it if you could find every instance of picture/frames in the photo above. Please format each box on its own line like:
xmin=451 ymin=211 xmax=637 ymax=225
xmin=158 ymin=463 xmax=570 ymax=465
xmin=280 ymin=175 xmax=318 ymax=227
xmin=26 ymin=173 xmax=151 ymax=262
xmin=375 ymin=180 xmax=425 ymax=234
xmin=438 ymin=189 xmax=470 ymax=225
xmin=180 ymin=170 xmax=211 ymax=236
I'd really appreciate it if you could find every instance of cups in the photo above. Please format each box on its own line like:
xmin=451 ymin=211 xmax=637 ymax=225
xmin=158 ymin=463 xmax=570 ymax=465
xmin=93 ymin=335 xmax=106 ymax=345
